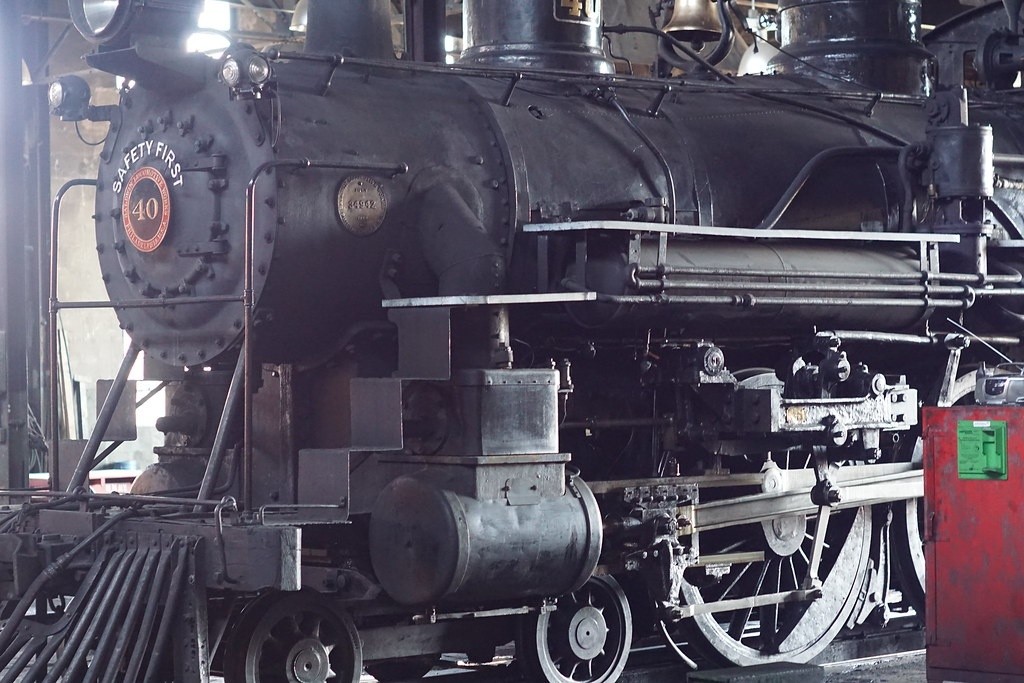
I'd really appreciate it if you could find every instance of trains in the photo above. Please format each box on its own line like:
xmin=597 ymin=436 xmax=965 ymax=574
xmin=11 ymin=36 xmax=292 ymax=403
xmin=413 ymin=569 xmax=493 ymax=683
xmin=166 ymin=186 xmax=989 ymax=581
xmin=2 ymin=2 xmax=1024 ymax=682
